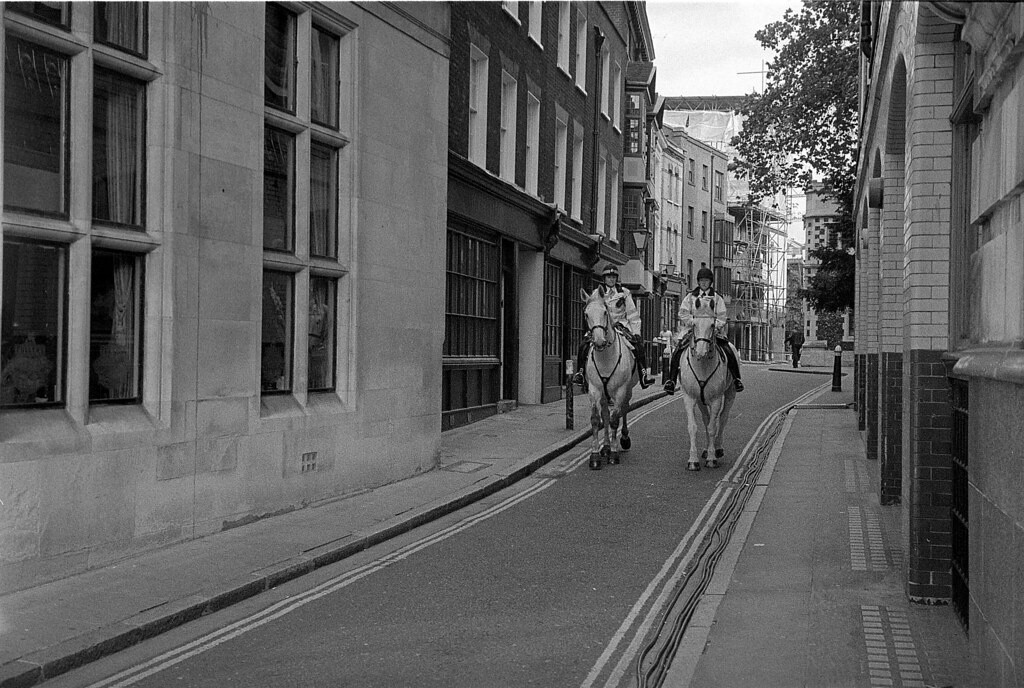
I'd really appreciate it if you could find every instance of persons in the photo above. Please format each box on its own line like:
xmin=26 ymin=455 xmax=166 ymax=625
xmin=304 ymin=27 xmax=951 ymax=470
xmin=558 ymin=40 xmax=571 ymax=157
xmin=572 ymin=263 xmax=655 ymax=389
xmin=664 ymin=268 xmax=744 ymax=395
xmin=784 ymin=326 xmax=805 ymax=368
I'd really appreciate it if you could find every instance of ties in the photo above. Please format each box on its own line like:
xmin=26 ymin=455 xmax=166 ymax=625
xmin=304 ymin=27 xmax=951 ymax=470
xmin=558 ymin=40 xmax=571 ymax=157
xmin=701 ymin=292 xmax=706 ymax=298
xmin=609 ymin=289 xmax=613 ymax=295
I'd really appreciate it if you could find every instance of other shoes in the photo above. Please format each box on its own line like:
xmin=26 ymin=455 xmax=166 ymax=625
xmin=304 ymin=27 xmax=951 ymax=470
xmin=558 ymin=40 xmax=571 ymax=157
xmin=639 ymin=369 xmax=655 ymax=389
xmin=663 ymin=382 xmax=674 ymax=395
xmin=573 ymin=368 xmax=584 ymax=383
xmin=733 ymin=378 xmax=744 ymax=391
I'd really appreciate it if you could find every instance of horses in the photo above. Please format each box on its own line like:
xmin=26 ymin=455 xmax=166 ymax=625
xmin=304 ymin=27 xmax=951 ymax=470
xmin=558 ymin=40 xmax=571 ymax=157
xmin=677 ymin=299 xmax=740 ymax=471
xmin=580 ymin=284 xmax=641 ymax=470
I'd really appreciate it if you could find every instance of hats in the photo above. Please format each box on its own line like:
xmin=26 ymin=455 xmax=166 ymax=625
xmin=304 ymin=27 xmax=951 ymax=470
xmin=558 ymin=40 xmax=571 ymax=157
xmin=602 ymin=263 xmax=619 ymax=277
xmin=697 ymin=268 xmax=714 ymax=284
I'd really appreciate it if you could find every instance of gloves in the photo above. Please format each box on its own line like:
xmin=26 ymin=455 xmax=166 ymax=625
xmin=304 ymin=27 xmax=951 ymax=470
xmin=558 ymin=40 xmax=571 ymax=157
xmin=635 ymin=334 xmax=642 ymax=342
